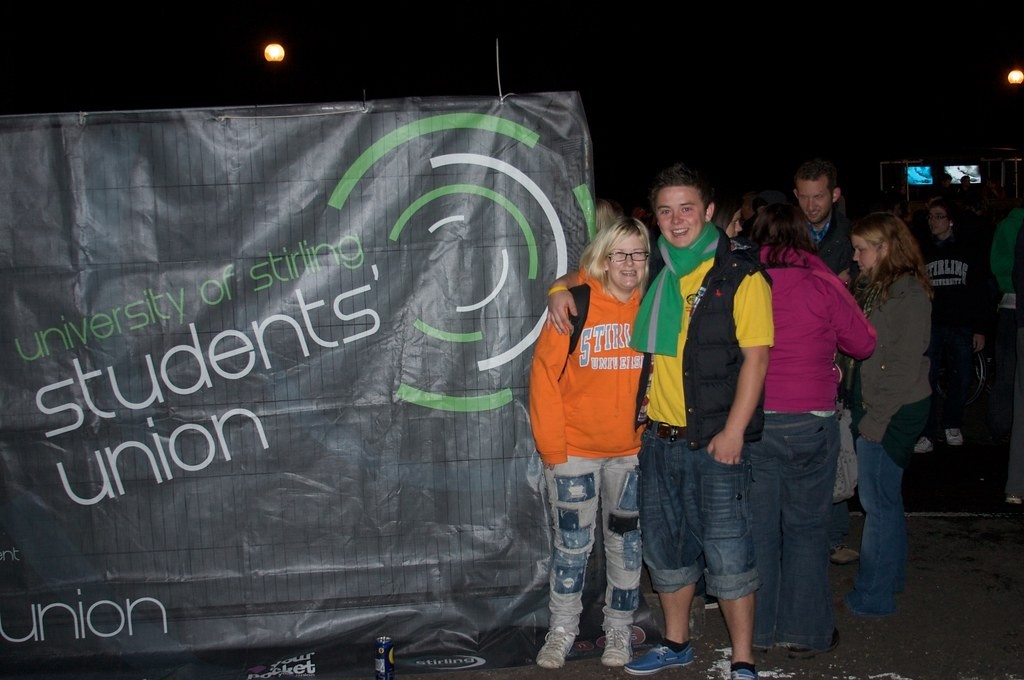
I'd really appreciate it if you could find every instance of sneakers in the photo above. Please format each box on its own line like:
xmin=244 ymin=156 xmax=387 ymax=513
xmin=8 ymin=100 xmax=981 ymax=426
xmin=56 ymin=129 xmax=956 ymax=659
xmin=623 ymin=641 xmax=694 ymax=675
xmin=945 ymin=429 xmax=963 ymax=446
xmin=536 ymin=627 xmax=578 ymax=668
xmin=600 ymin=626 xmax=633 ymax=666
xmin=913 ymin=437 xmax=934 ymax=454
xmin=731 ymin=669 xmax=759 ymax=680
xmin=831 ymin=548 xmax=859 ymax=563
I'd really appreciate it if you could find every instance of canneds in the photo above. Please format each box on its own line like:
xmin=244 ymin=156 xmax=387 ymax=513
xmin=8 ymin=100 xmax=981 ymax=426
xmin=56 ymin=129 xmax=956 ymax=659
xmin=373 ymin=636 xmax=395 ymax=680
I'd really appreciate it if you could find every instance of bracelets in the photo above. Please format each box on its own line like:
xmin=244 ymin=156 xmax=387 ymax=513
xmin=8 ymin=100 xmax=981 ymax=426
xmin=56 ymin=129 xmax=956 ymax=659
xmin=548 ymin=286 xmax=568 ymax=295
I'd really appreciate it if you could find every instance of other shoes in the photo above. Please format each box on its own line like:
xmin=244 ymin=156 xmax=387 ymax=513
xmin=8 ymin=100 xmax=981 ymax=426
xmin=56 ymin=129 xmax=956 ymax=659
xmin=703 ymin=596 xmax=719 ymax=610
xmin=789 ymin=628 xmax=839 ymax=658
xmin=1005 ymin=494 xmax=1023 ymax=504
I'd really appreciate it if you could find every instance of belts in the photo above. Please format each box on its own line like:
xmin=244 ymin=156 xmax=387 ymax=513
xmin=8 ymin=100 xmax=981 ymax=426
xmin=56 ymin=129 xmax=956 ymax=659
xmin=646 ymin=417 xmax=687 ymax=439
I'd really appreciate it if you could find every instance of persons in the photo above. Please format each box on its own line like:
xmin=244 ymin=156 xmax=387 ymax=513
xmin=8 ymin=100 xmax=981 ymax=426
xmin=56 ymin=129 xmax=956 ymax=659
xmin=593 ymin=157 xmax=1024 ymax=657
xmin=529 ymin=218 xmax=651 ymax=669
xmin=546 ymin=166 xmax=776 ymax=680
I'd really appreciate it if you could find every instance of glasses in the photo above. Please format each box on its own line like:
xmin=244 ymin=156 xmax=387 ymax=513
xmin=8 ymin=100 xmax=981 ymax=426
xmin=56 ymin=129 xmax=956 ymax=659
xmin=608 ymin=251 xmax=649 ymax=262
xmin=926 ymin=214 xmax=948 ymax=219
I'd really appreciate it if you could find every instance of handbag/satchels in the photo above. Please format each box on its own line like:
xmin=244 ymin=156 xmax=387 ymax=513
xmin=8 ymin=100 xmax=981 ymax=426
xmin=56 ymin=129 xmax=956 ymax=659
xmin=832 ymin=409 xmax=857 ymax=504
